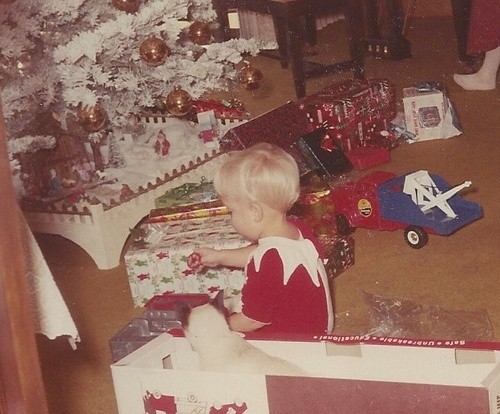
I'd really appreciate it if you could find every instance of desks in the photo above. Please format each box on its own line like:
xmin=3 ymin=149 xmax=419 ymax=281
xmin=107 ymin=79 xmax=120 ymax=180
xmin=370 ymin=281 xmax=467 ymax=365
xmin=215 ymin=0 xmax=368 ymax=99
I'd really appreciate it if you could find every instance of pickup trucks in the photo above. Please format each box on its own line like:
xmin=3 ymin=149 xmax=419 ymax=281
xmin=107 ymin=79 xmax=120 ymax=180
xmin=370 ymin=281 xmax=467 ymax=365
xmin=330 ymin=169 xmax=483 ymax=249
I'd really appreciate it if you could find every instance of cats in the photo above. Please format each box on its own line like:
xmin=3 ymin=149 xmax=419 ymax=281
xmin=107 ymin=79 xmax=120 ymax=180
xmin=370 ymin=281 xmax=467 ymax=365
xmin=174 ymin=289 xmax=313 ymax=377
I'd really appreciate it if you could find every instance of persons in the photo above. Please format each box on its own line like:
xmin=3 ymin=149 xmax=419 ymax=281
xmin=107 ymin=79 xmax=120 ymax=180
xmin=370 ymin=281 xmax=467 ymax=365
xmin=187 ymin=142 xmax=335 ymax=335
xmin=453 ymin=0 xmax=500 ymax=90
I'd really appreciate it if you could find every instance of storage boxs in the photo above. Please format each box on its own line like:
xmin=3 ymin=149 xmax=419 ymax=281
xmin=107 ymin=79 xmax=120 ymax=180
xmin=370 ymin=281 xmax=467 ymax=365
xmin=125 ymin=182 xmax=270 ymax=321
xmin=112 ymin=333 xmax=499 ymax=413
xmin=305 ymin=81 xmax=445 ymax=153
